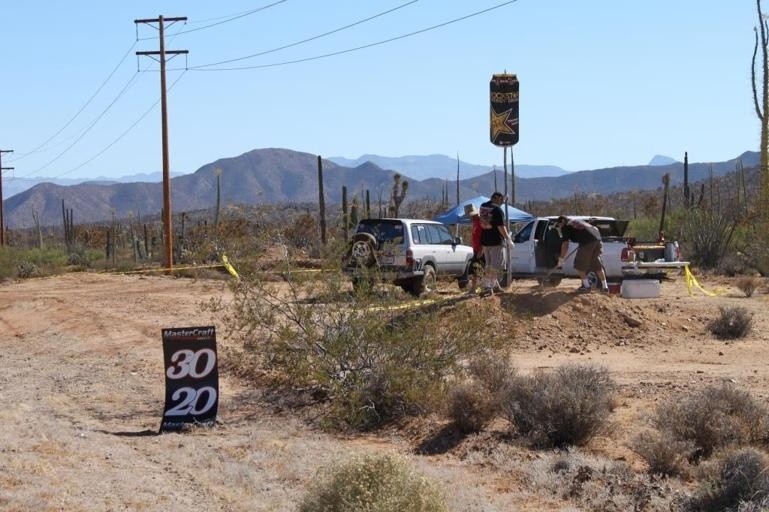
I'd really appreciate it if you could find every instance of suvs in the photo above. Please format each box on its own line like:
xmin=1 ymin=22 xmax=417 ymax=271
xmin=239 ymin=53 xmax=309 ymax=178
xmin=340 ymin=217 xmax=479 ymax=299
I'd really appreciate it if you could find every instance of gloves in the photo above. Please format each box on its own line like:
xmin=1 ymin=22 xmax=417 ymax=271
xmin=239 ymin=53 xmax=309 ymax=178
xmin=559 ymin=257 xmax=564 ymax=267
xmin=507 ymin=239 xmax=514 ymax=249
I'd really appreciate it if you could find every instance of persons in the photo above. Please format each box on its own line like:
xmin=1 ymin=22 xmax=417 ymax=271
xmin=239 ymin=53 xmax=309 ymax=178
xmin=462 ymin=203 xmax=485 ymax=294
xmin=479 ymin=192 xmax=516 ymax=296
xmin=556 ymin=215 xmax=609 ymax=293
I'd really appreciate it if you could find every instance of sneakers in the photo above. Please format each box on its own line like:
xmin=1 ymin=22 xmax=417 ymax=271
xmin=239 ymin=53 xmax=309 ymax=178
xmin=468 ymin=285 xmax=504 ymax=296
xmin=600 ymin=288 xmax=609 ymax=295
xmin=578 ymin=286 xmax=591 ymax=293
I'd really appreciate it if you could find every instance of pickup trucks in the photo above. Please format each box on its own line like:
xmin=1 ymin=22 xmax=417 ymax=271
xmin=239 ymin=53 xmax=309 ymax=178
xmin=482 ymin=212 xmax=690 ymax=293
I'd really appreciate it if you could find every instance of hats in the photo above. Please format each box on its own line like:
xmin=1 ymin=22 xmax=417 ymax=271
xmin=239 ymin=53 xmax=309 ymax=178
xmin=462 ymin=203 xmax=479 ymax=218
